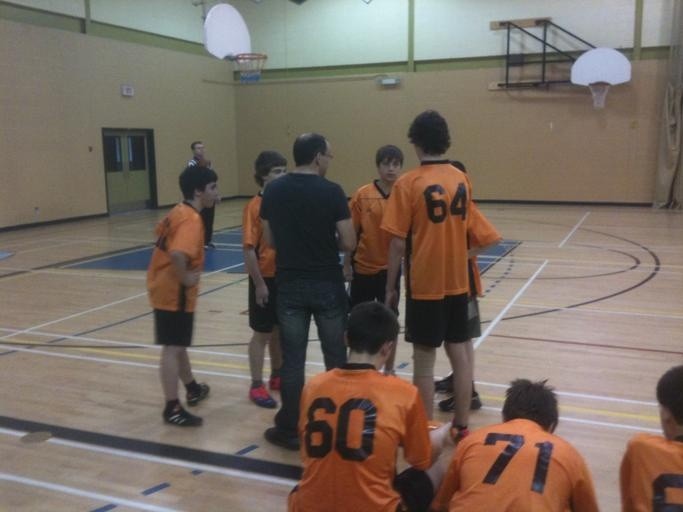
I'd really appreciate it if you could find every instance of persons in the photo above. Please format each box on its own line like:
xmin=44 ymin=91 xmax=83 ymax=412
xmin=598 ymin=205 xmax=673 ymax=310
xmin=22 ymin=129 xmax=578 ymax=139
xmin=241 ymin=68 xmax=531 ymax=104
xmin=285 ymin=301 xmax=453 ymax=511
xmin=147 ymin=165 xmax=219 ymax=426
xmin=187 ymin=141 xmax=221 ymax=249
xmin=429 ymin=376 xmax=600 ymax=512
xmin=620 ymin=364 xmax=683 ymax=512
xmin=243 ymin=111 xmax=503 ymax=450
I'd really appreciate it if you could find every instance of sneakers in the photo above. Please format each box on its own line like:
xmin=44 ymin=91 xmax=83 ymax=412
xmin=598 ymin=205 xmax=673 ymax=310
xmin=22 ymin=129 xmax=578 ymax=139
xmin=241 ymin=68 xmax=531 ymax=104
xmin=450 ymin=418 xmax=469 ymax=445
xmin=438 ymin=391 xmax=481 ymax=412
xmin=186 ymin=383 xmax=209 ymax=406
xmin=162 ymin=403 xmax=202 ymax=427
xmin=434 ymin=373 xmax=474 ymax=393
xmin=249 ymin=375 xmax=302 ymax=450
